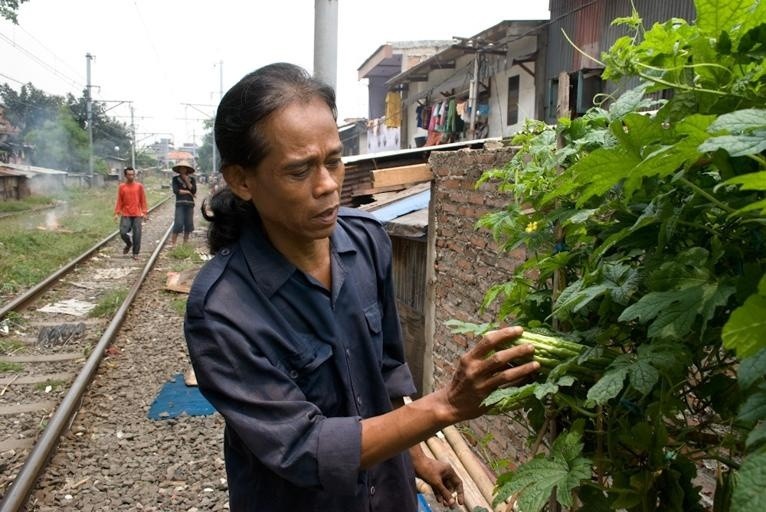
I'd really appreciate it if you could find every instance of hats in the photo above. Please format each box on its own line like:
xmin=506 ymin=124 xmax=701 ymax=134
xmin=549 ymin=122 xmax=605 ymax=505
xmin=172 ymin=160 xmax=195 ymax=174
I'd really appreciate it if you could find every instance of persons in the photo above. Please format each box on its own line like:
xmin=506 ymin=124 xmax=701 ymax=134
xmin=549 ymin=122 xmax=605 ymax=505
xmin=114 ymin=167 xmax=152 ymax=262
xmin=183 ymin=61 xmax=542 ymax=511
xmin=168 ymin=160 xmax=198 ymax=250
